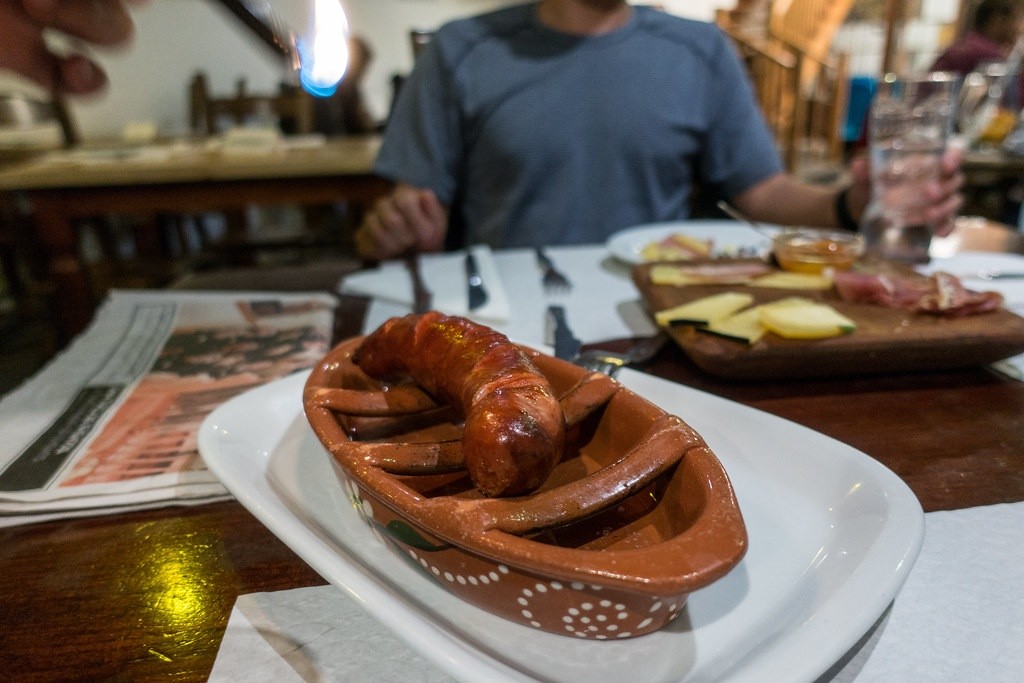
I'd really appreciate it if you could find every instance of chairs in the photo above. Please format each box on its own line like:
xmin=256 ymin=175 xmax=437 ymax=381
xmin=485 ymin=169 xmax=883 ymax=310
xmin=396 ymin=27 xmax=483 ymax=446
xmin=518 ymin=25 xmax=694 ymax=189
xmin=180 ymin=72 xmax=323 ymax=267
xmin=712 ymin=5 xmax=850 ymax=175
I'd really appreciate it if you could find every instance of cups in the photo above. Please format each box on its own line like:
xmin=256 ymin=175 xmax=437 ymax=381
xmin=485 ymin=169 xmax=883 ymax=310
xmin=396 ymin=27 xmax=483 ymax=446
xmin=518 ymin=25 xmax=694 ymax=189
xmin=773 ymin=233 xmax=860 ymax=275
xmin=868 ymin=72 xmax=956 ymax=226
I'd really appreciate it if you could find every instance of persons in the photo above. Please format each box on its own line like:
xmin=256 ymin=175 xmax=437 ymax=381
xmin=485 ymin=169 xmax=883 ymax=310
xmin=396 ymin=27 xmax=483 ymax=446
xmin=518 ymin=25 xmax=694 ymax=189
xmin=355 ymin=1 xmax=964 ymax=261
xmin=916 ymin=0 xmax=1024 ymax=106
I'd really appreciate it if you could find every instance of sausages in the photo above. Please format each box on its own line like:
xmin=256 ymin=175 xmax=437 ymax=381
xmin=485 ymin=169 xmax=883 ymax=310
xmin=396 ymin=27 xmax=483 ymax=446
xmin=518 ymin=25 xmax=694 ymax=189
xmin=352 ymin=310 xmax=564 ymax=498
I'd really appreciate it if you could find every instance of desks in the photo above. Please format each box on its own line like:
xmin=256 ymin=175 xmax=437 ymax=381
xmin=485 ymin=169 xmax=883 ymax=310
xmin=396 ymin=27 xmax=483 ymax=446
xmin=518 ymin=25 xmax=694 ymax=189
xmin=2 ymin=134 xmax=387 ymax=335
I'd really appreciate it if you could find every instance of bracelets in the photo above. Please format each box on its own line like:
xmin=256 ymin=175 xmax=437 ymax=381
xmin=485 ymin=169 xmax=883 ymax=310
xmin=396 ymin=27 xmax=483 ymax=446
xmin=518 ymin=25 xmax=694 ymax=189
xmin=834 ymin=185 xmax=855 ymax=235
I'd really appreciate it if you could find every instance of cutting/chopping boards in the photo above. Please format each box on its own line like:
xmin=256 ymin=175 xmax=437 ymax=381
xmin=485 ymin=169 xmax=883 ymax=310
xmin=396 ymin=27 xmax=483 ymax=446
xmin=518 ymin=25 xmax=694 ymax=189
xmin=631 ymin=258 xmax=1024 ymax=376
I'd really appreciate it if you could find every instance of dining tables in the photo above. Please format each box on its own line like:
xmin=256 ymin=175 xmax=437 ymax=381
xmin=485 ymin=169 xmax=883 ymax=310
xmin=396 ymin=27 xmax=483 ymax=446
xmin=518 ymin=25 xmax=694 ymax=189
xmin=0 ymin=270 xmax=1024 ymax=683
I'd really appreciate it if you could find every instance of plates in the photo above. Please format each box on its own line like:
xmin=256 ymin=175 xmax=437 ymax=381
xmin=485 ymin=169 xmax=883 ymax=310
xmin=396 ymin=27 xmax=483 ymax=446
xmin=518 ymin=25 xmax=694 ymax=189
xmin=606 ymin=218 xmax=782 ymax=265
xmin=196 ymin=363 xmax=926 ymax=683
xmin=301 ymin=334 xmax=749 ymax=597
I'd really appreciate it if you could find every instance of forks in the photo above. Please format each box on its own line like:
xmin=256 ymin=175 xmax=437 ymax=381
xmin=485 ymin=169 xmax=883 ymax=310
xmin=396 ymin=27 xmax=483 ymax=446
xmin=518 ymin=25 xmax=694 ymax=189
xmin=577 ymin=335 xmax=668 ymax=377
xmin=536 ymin=246 xmax=571 ymax=296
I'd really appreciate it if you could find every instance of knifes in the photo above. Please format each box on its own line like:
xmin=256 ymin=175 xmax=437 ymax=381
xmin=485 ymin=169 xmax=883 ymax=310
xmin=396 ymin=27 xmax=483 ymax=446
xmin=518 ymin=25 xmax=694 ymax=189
xmin=550 ymin=305 xmax=581 ymax=364
xmin=466 ymin=254 xmax=488 ymax=310
